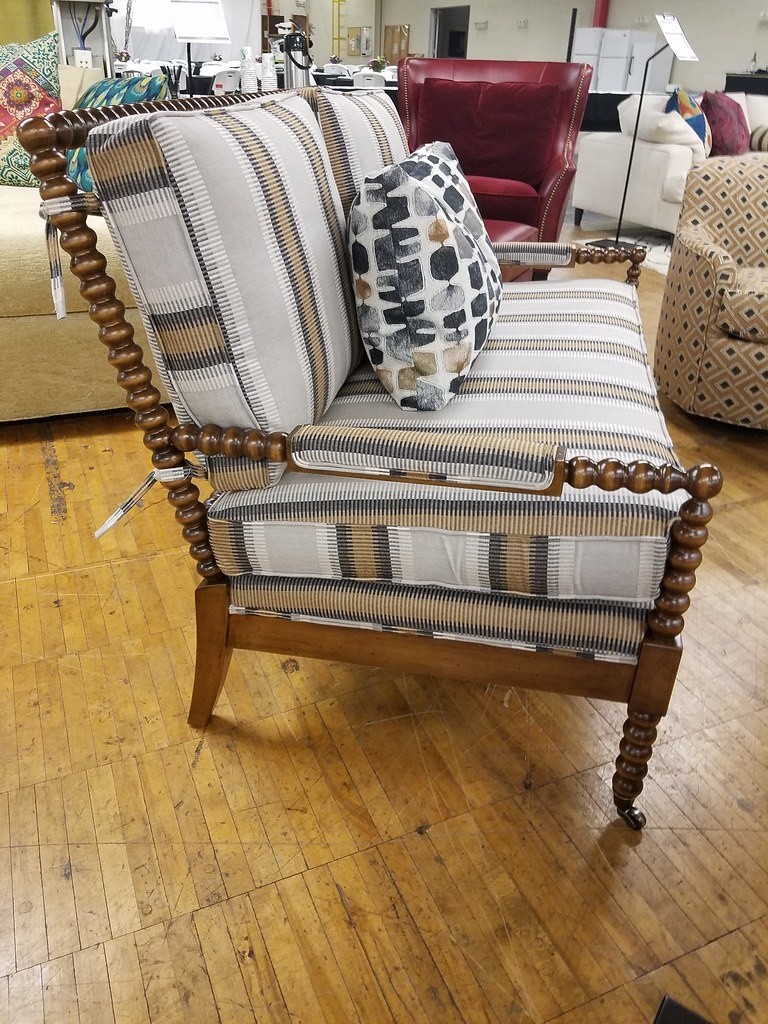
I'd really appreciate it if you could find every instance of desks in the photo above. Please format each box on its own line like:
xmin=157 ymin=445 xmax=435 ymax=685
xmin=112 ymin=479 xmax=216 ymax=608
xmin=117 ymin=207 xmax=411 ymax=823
xmin=323 ymin=86 xmax=399 ymax=111
xmin=325 ymin=79 xmax=397 ymax=85
xmin=276 ymin=71 xmax=346 ymax=89
xmin=722 ymin=73 xmax=768 ymax=97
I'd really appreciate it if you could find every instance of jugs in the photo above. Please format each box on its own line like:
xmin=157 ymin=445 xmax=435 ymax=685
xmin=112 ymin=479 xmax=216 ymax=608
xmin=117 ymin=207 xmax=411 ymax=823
xmin=283 ymin=34 xmax=313 ymax=88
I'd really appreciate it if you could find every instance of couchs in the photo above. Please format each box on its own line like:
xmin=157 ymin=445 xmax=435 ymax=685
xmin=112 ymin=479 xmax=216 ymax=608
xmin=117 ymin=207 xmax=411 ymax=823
xmin=652 ymin=152 xmax=768 ymax=430
xmin=572 ymin=92 xmax=768 ymax=245
xmin=16 ymin=85 xmax=724 ymax=830
xmin=0 ymin=64 xmax=173 ymax=423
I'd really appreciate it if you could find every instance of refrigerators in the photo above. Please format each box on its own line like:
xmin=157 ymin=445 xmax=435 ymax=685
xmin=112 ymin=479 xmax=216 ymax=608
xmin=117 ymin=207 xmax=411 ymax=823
xmin=596 ymin=29 xmax=657 ymax=93
xmin=571 ymin=28 xmax=608 ymax=94
xmin=626 ymin=41 xmax=673 ymax=92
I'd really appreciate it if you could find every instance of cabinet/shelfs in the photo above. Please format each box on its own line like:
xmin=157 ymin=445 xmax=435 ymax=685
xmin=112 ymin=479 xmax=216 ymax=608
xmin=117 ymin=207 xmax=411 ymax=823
xmin=573 ymin=28 xmax=674 ymax=92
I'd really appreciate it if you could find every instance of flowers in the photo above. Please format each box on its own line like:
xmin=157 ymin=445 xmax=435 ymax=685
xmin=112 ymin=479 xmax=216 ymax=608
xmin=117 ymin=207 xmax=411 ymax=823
xmin=255 ymin=57 xmax=262 ymax=63
xmin=211 ymin=53 xmax=222 ymax=61
xmin=114 ymin=51 xmax=131 ymax=62
xmin=329 ymin=55 xmax=343 ymax=64
xmin=368 ymin=56 xmax=390 ymax=72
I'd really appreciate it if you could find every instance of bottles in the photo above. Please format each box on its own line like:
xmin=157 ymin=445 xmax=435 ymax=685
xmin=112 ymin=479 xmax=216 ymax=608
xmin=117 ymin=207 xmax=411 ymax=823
xmin=214 ymin=83 xmax=223 ymax=96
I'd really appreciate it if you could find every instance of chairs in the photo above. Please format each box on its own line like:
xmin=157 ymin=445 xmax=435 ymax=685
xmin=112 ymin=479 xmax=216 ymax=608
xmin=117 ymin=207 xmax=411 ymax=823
xmin=396 ymin=57 xmax=593 ymax=282
xmin=114 ymin=58 xmax=398 ymax=95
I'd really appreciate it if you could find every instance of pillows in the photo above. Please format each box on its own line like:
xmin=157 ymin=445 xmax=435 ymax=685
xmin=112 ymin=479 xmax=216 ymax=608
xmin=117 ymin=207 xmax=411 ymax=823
xmin=64 ymin=75 xmax=170 ymax=196
xmin=749 ymin=126 xmax=768 ymax=152
xmin=700 ymin=90 xmax=750 ymax=156
xmin=416 ymin=77 xmax=559 ymax=186
xmin=0 ymin=31 xmax=60 ymax=189
xmin=345 ymin=139 xmax=506 ymax=411
xmin=665 ymin=87 xmax=712 ymax=159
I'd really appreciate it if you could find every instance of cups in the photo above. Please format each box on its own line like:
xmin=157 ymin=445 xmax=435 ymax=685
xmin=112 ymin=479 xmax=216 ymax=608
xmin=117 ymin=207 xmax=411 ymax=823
xmin=241 ymin=47 xmax=258 ymax=94
xmin=261 ymin=53 xmax=277 ymax=91
xmin=166 ymin=81 xmax=180 ymax=100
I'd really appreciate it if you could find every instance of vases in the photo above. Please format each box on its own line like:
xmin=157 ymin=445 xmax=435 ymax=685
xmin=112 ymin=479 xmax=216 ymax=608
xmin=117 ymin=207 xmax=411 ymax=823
xmin=373 ymin=67 xmax=381 ymax=73
